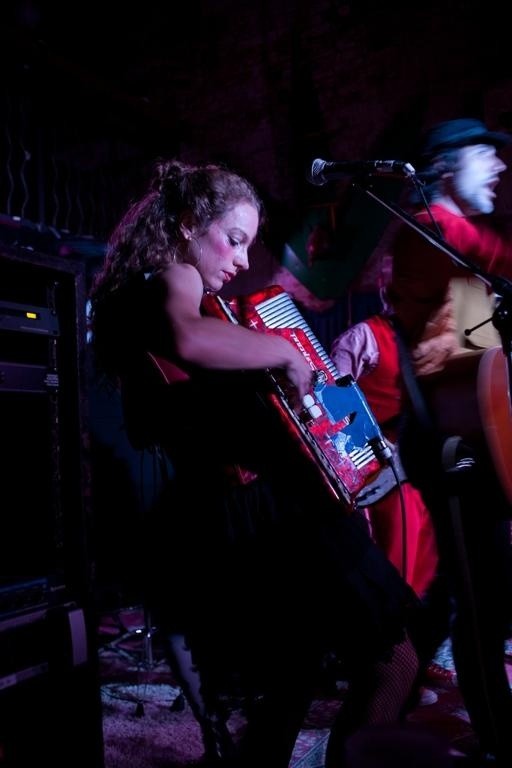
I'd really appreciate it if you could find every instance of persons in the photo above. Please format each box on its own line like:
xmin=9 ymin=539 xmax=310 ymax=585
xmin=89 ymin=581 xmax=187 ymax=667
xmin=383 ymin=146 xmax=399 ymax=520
xmin=87 ymin=160 xmax=421 ymax=766
xmin=322 ymin=202 xmax=511 ymax=768
xmin=372 ymin=114 xmax=511 ymax=318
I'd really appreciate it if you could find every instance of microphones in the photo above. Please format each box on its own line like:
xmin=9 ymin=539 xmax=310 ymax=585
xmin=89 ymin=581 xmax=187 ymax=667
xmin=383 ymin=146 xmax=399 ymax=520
xmin=305 ymin=156 xmax=412 ymax=187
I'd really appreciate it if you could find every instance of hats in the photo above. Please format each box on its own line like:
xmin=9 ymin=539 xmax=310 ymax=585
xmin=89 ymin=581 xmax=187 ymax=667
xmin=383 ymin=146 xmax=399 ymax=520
xmin=423 ymin=118 xmax=512 ymax=157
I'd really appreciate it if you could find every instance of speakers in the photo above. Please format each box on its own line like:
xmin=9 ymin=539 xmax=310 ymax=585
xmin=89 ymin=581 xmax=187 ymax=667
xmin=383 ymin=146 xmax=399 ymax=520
xmin=1 ymin=257 xmax=94 ymax=614
xmin=0 ymin=600 xmax=110 ymax=765
